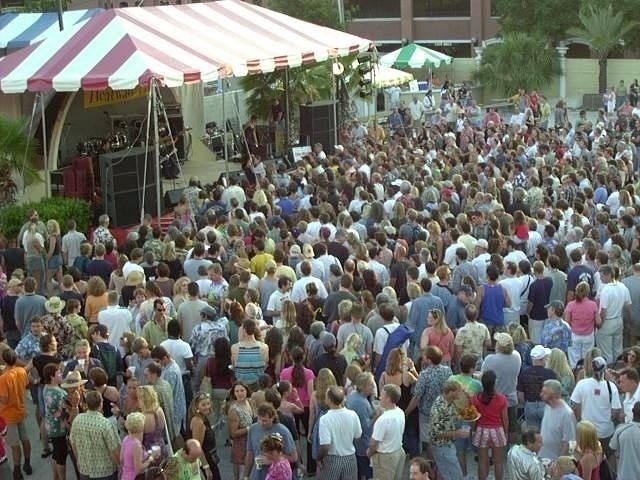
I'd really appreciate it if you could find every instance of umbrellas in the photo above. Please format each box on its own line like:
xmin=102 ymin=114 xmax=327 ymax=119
xmin=378 ymin=43 xmax=454 ymax=81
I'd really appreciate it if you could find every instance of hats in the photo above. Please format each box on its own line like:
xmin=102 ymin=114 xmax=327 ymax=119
xmin=494 ymin=332 xmax=513 ymax=347
xmin=314 ymin=143 xmax=322 ymax=148
xmin=544 ymin=300 xmax=565 ymax=313
xmin=489 ymin=204 xmax=504 ymax=214
xmin=320 ymin=331 xmax=337 ymax=347
xmin=288 ymin=229 xmax=316 ymax=258
xmin=265 ymin=260 xmax=276 ymax=270
xmin=473 ymin=239 xmax=490 ymax=249
xmin=530 ymin=345 xmax=551 ymax=361
xmin=234 ymin=258 xmax=250 ymax=272
xmin=199 ymin=307 xmax=217 ymax=318
xmin=60 ymin=370 xmax=88 ymax=388
xmin=301 ymin=155 xmax=310 ymax=160
xmin=190 ymin=175 xmax=199 ymax=183
xmin=321 ymin=227 xmax=330 ymax=237
xmin=310 ymin=320 xmax=324 ymax=337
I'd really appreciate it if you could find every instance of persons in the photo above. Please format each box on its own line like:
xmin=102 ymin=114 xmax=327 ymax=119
xmin=1 ymin=71 xmax=640 ymax=479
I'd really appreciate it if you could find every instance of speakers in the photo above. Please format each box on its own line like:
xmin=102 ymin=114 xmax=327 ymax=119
xmin=165 ymin=189 xmax=186 ymax=208
xmin=216 ymin=170 xmax=247 ymax=184
xmin=300 ymin=101 xmax=339 ymax=152
xmin=99 ymin=153 xmax=138 ymax=228
xmin=119 ymin=145 xmax=166 ymax=222
xmin=289 ymin=146 xmax=312 ymax=163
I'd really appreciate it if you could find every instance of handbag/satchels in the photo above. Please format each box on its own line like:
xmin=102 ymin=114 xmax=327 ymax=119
xmin=45 ymin=296 xmax=66 ymax=314
xmin=27 ymin=367 xmax=40 ymax=385
xmin=125 ymin=270 xmax=145 ymax=286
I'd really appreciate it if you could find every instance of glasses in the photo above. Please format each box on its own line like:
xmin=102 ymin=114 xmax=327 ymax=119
xmin=156 ymin=308 xmax=166 ymax=312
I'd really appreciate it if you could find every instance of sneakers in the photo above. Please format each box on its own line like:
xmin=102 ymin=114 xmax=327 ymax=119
xmin=13 ymin=471 xmax=24 ymax=480
xmin=41 ymin=448 xmax=53 ymax=458
xmin=23 ymin=463 xmax=33 ymax=475
